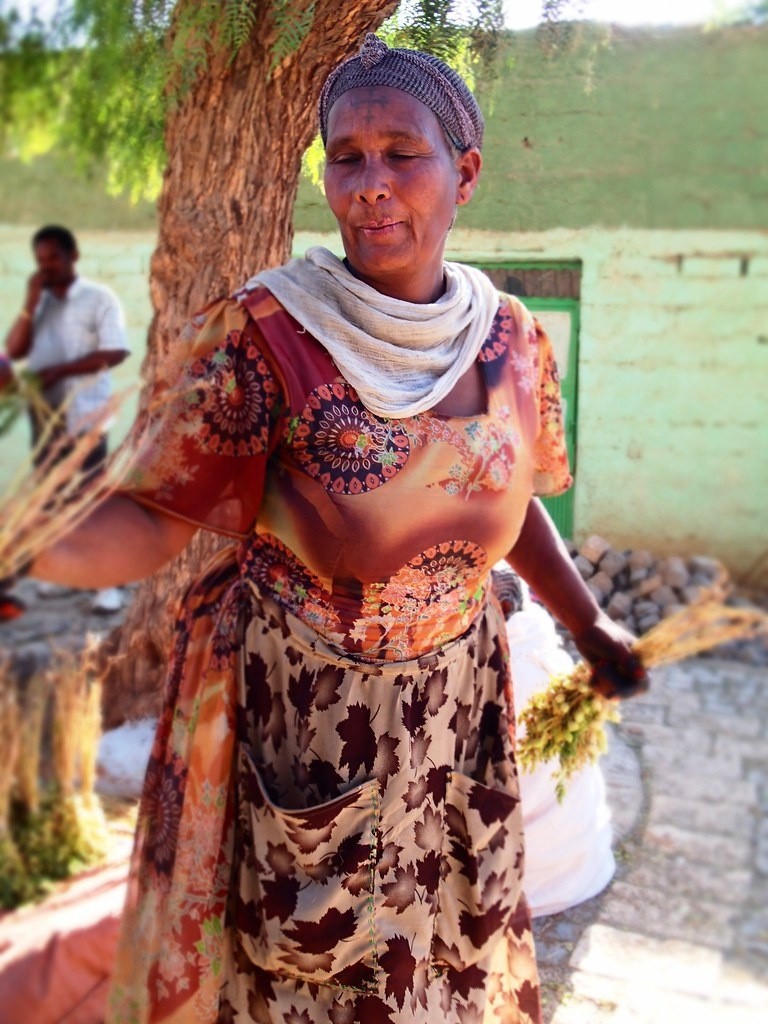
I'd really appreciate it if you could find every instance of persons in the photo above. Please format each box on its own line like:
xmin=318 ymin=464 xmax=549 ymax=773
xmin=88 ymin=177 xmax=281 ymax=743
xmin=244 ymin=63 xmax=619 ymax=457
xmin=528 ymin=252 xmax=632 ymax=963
xmin=4 ymin=224 xmax=132 ymax=614
xmin=0 ymin=33 xmax=649 ymax=1024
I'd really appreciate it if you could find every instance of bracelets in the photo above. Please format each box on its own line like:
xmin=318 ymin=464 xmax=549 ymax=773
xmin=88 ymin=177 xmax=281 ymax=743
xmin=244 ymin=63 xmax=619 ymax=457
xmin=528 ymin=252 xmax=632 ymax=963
xmin=20 ymin=311 xmax=36 ymax=322
